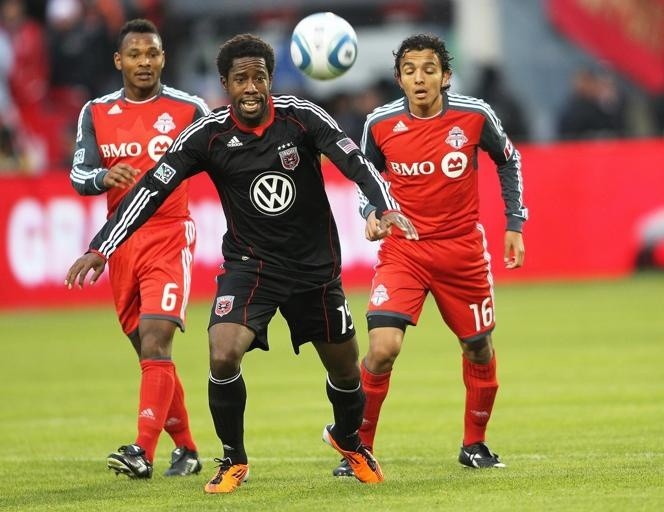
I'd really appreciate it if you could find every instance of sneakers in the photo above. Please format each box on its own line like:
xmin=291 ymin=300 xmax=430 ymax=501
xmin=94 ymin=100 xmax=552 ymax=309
xmin=321 ymin=423 xmax=385 ymax=485
xmin=107 ymin=444 xmax=153 ymax=480
xmin=457 ymin=441 xmax=505 ymax=470
xmin=204 ymin=462 xmax=250 ymax=494
xmin=163 ymin=446 xmax=201 ymax=477
xmin=332 ymin=456 xmax=356 ymax=477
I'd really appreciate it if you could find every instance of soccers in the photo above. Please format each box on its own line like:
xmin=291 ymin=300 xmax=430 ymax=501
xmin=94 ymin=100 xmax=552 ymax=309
xmin=291 ymin=11 xmax=358 ymax=81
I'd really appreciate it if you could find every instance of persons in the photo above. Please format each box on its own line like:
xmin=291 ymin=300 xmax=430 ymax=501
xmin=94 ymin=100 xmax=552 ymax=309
xmin=70 ymin=18 xmax=211 ymax=478
xmin=65 ymin=31 xmax=420 ymax=494
xmin=331 ymin=33 xmax=528 ymax=478
